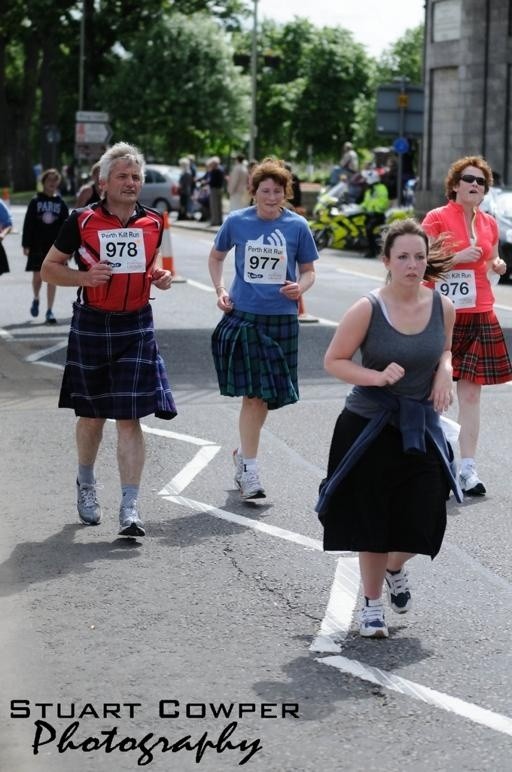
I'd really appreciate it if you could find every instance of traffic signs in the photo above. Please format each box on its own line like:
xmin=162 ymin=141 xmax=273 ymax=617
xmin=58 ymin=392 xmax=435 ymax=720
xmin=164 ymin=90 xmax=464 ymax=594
xmin=75 ymin=110 xmax=110 ymax=122
xmin=75 ymin=123 xmax=115 ymax=145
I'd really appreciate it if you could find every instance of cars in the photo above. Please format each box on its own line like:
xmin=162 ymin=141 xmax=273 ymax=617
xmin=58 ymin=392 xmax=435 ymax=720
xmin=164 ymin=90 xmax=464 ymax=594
xmin=74 ymin=162 xmax=204 ymax=220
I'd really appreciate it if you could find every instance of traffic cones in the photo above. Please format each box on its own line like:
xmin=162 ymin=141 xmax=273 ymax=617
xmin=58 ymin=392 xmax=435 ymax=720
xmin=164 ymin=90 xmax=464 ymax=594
xmin=1 ymin=185 xmax=18 ymax=236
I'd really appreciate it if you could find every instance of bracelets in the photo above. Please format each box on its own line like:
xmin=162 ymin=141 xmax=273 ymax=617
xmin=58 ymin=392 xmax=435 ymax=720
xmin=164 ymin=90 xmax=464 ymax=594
xmin=216 ymin=287 xmax=226 ymax=294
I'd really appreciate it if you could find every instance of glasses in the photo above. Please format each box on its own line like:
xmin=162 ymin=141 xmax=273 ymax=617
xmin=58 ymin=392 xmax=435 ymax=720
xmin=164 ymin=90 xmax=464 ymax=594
xmin=458 ymin=175 xmax=486 ymax=186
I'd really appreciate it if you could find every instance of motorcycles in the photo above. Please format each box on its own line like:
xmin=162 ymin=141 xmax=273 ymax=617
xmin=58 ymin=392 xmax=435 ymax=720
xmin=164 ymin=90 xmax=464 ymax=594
xmin=298 ymin=181 xmax=386 ymax=254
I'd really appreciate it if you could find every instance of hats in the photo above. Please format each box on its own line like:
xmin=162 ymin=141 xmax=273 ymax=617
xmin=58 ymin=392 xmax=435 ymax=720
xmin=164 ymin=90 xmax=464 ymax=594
xmin=361 ymin=170 xmax=381 ymax=185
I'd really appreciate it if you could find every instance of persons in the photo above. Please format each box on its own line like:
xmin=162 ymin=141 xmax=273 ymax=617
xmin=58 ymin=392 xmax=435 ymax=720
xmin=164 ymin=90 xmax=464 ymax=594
xmin=312 ymin=219 xmax=467 ymax=641
xmin=20 ymin=168 xmax=71 ymax=324
xmin=412 ymin=154 xmax=512 ymax=500
xmin=206 ymin=156 xmax=321 ymax=501
xmin=0 ymin=200 xmax=15 ymax=278
xmin=74 ymin=161 xmax=101 ymax=209
xmin=37 ymin=140 xmax=179 ymax=540
xmin=174 ymin=140 xmax=392 ymax=260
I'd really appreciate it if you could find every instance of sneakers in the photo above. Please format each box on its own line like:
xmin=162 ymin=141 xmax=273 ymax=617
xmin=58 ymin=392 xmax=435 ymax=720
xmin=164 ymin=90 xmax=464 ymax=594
xmin=118 ymin=505 xmax=145 ymax=536
xmin=360 ymin=595 xmax=389 ymax=639
xmin=233 ymin=448 xmax=267 ymax=498
xmin=458 ymin=466 xmax=487 ymax=493
xmin=46 ymin=310 xmax=56 ymax=323
xmin=385 ymin=566 xmax=412 ymax=614
xmin=77 ymin=475 xmax=103 ymax=524
xmin=32 ymin=300 xmax=39 ymax=317
